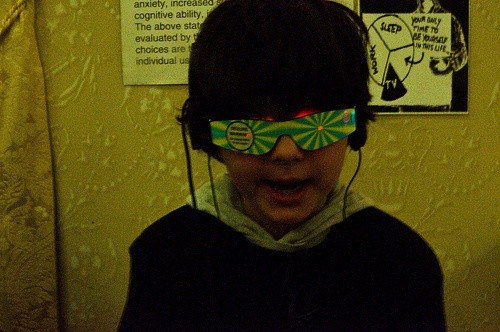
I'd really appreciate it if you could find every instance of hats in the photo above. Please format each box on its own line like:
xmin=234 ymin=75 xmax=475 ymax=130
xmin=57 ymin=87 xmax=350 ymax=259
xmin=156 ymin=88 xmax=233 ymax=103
xmin=184 ymin=1 xmax=372 ymax=113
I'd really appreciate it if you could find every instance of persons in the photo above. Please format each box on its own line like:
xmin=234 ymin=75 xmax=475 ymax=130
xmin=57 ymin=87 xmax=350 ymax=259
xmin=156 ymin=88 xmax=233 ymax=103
xmin=114 ymin=2 xmax=449 ymax=332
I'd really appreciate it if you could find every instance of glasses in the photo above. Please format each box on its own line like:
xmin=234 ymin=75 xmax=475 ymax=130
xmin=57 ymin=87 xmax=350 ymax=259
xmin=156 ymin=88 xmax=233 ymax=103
xmin=205 ymin=108 xmax=360 ymax=157
